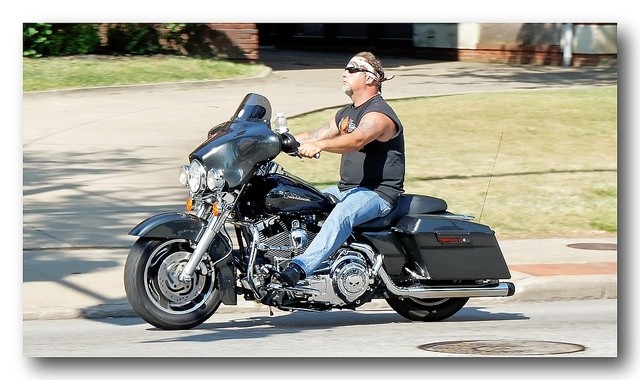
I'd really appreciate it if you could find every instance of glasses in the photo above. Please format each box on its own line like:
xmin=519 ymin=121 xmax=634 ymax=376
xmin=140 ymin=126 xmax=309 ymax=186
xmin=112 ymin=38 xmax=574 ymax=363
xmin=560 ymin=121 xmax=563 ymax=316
xmin=344 ymin=67 xmax=378 ymax=78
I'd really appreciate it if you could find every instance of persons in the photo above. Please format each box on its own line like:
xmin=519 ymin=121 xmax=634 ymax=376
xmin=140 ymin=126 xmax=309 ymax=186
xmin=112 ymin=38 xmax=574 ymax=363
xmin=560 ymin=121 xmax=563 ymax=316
xmin=277 ymin=51 xmax=406 ymax=289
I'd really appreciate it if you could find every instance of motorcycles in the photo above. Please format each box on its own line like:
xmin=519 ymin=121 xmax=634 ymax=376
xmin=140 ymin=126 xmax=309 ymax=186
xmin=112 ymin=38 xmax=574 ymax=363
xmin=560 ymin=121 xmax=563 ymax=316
xmin=124 ymin=93 xmax=515 ymax=330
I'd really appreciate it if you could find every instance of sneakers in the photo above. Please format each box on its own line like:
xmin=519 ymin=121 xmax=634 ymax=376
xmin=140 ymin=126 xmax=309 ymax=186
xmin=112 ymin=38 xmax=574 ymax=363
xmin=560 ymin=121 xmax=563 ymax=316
xmin=282 ymin=266 xmax=298 ymax=287
